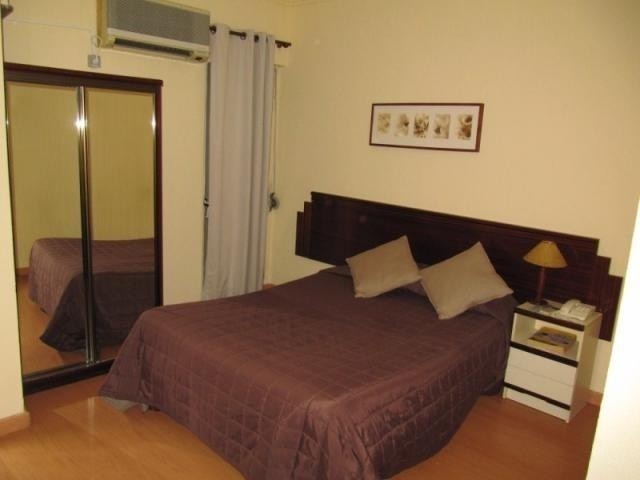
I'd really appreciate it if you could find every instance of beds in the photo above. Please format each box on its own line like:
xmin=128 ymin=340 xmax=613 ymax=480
xmin=95 ymin=190 xmax=625 ymax=479
xmin=26 ymin=237 xmax=156 ymax=351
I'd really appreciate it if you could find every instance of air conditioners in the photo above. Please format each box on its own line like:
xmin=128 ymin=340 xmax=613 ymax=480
xmin=96 ymin=0 xmax=210 ymax=63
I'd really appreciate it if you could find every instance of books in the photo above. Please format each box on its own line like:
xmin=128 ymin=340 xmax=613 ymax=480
xmin=529 ymin=327 xmax=577 ymax=356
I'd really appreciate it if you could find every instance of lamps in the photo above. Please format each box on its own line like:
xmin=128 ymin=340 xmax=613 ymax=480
xmin=522 ymin=241 xmax=567 ymax=306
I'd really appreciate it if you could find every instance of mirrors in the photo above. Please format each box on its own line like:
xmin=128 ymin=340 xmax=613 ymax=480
xmin=4 ymin=59 xmax=164 ymax=398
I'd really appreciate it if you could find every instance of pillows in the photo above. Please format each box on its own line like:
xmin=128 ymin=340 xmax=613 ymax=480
xmin=420 ymin=241 xmax=515 ymax=320
xmin=344 ymin=235 xmax=422 ymax=298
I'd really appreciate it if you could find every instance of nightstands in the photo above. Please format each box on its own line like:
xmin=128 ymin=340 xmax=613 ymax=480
xmin=501 ymin=300 xmax=602 ymax=423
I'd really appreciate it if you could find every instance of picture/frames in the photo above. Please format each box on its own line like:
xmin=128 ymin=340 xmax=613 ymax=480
xmin=369 ymin=102 xmax=484 ymax=152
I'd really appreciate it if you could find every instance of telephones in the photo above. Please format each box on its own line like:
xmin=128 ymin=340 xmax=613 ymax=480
xmin=559 ymin=298 xmax=597 ymax=321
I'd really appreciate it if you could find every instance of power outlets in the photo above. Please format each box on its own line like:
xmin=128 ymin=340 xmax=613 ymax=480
xmin=88 ymin=55 xmax=101 ymax=67
